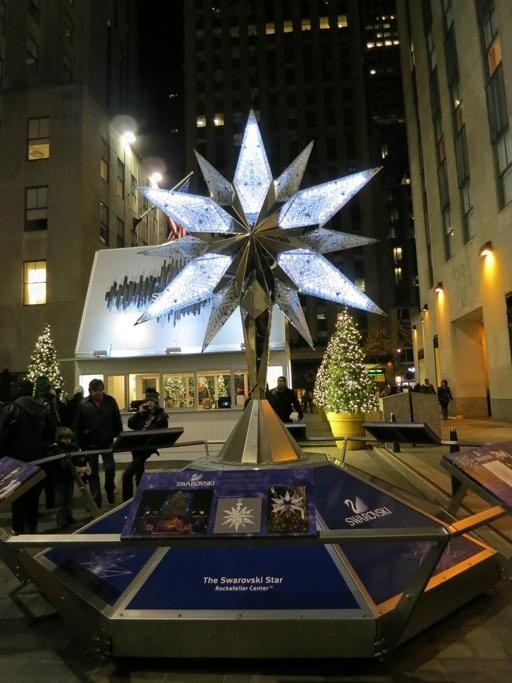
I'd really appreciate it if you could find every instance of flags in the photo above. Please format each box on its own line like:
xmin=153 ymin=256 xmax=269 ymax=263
xmin=163 ymin=171 xmax=195 ymax=246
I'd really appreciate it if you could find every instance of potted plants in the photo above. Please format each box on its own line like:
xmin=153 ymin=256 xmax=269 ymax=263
xmin=311 ymin=306 xmax=378 ymax=450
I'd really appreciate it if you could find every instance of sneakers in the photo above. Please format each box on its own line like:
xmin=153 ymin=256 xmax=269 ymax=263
xmin=108 ymin=491 xmax=114 ymax=504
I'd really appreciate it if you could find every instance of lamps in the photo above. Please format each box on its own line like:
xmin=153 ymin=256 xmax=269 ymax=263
xmin=411 ymin=241 xmax=492 ymax=330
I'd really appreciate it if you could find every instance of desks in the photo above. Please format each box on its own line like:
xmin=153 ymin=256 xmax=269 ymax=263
xmin=235 ymin=394 xmax=244 ymax=405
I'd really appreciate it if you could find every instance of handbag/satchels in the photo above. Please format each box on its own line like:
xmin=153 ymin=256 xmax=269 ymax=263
xmin=76 ymin=462 xmax=92 ymax=478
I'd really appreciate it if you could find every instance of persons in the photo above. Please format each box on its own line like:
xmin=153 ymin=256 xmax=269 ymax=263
xmin=122 ymin=392 xmax=169 ymax=502
xmin=370 ymin=378 xmax=454 ymax=420
xmin=244 ymin=376 xmax=314 ymax=422
xmin=0 ymin=367 xmax=123 ymax=536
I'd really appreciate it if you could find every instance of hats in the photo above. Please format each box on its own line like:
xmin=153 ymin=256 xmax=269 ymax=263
xmin=73 ymin=384 xmax=84 ymax=395
